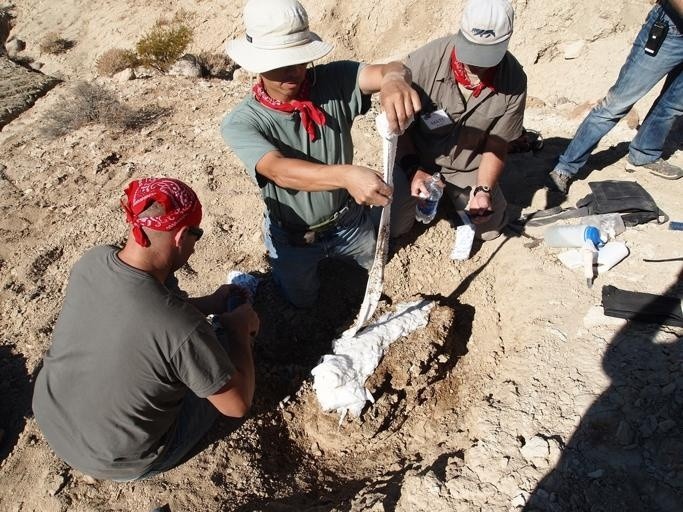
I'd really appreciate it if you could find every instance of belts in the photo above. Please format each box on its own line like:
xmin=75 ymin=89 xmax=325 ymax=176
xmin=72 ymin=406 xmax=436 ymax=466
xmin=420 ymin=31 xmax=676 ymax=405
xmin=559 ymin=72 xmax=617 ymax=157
xmin=269 ymin=214 xmax=337 ymax=234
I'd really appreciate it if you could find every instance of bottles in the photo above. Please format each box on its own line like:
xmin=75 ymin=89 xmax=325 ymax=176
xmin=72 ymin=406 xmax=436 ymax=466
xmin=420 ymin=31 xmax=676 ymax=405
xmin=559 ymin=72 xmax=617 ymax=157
xmin=414 ymin=171 xmax=443 ymax=225
xmin=543 ymin=225 xmax=604 ymax=248
xmin=224 ymin=289 xmax=254 ymax=349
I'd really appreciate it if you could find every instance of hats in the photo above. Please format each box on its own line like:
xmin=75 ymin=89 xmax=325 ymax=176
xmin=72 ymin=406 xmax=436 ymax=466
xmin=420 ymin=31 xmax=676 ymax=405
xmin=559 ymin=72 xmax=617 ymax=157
xmin=455 ymin=0 xmax=513 ymax=67
xmin=224 ymin=0 xmax=334 ymax=74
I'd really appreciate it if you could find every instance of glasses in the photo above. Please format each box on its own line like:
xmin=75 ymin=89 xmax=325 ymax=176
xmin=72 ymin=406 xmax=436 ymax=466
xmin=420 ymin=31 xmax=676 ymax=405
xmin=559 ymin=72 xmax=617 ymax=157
xmin=188 ymin=227 xmax=203 ymax=240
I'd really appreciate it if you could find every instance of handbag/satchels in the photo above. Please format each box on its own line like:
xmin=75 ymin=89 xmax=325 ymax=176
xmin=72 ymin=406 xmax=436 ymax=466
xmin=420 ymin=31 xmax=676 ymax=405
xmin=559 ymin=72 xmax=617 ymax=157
xmin=575 ymin=181 xmax=668 ymax=227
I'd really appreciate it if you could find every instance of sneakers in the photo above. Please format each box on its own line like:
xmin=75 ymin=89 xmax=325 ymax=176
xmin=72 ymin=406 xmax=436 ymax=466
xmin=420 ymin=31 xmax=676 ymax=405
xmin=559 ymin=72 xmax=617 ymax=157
xmin=543 ymin=170 xmax=570 ymax=196
xmin=624 ymin=157 xmax=682 ymax=180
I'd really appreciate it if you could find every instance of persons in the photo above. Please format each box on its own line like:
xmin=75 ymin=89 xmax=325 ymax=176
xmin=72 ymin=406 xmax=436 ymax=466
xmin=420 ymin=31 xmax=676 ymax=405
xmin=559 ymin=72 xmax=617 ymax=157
xmin=29 ymin=176 xmax=265 ymax=484
xmin=543 ymin=0 xmax=683 ymax=201
xmin=217 ymin=0 xmax=425 ymax=312
xmin=382 ymin=0 xmax=528 ymax=242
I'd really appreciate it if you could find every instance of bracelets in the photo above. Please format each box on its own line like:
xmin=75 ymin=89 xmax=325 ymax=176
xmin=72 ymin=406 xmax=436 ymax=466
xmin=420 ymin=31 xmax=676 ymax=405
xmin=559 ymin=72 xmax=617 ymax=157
xmin=473 ymin=184 xmax=495 ymax=198
